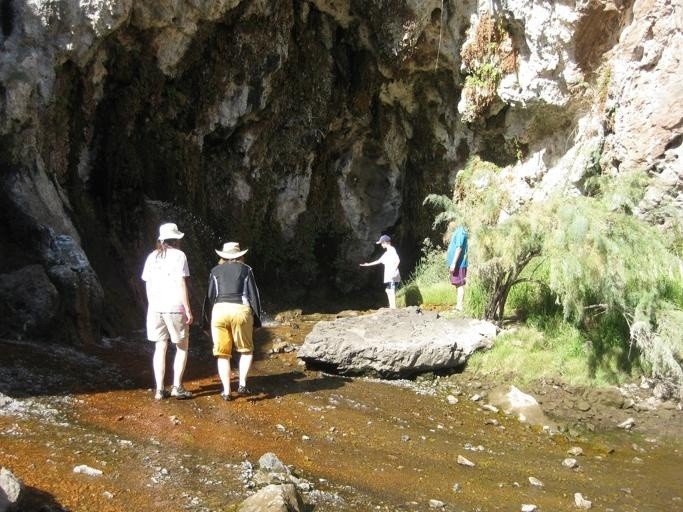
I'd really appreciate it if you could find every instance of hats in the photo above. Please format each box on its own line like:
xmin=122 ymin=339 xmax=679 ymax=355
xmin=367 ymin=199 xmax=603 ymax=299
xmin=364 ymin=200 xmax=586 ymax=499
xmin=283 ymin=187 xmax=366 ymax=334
xmin=376 ymin=234 xmax=391 ymax=244
xmin=215 ymin=241 xmax=249 ymax=259
xmin=157 ymin=223 xmax=185 ymax=241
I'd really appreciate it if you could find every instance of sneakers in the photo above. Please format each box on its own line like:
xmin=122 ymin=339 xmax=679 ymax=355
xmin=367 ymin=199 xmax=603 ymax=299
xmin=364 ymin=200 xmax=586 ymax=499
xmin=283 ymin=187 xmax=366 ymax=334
xmin=155 ymin=385 xmax=192 ymax=400
xmin=221 ymin=385 xmax=252 ymax=401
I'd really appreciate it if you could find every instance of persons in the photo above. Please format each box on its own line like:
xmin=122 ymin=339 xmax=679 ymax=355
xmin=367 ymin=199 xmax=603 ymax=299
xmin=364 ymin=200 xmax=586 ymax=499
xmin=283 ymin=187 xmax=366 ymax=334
xmin=359 ymin=235 xmax=401 ymax=308
xmin=208 ymin=241 xmax=262 ymax=401
xmin=142 ymin=222 xmax=194 ymax=399
xmin=446 ymin=218 xmax=468 ymax=313
